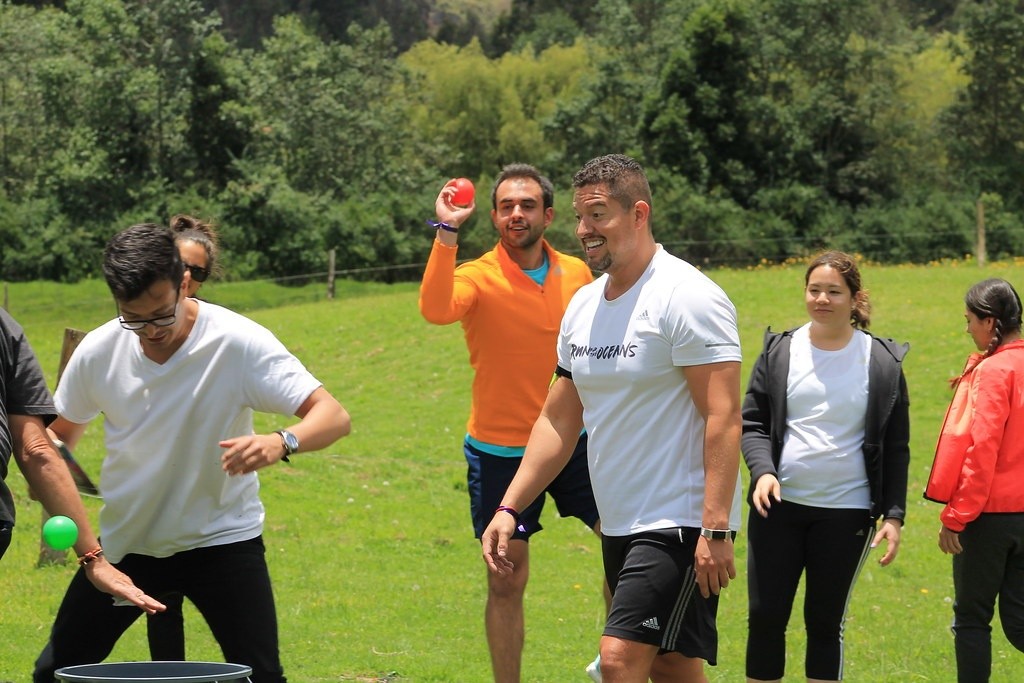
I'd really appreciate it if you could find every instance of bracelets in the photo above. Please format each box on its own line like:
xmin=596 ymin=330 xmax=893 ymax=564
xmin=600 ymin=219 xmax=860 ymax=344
xmin=427 ymin=221 xmax=459 ymax=233
xmin=496 ymin=506 xmax=519 ymax=519
xmin=79 ymin=548 xmax=103 ymax=564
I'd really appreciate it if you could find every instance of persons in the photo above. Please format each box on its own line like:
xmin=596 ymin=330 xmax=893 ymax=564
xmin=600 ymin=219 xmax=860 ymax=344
xmin=740 ymin=251 xmax=910 ymax=683
xmin=418 ymin=163 xmax=614 ymax=683
xmin=32 ymin=223 xmax=351 ymax=683
xmin=923 ymin=277 xmax=1024 ymax=683
xmin=0 ymin=306 xmax=166 ymax=614
xmin=144 ymin=214 xmax=216 ymax=661
xmin=481 ymin=154 xmax=742 ymax=683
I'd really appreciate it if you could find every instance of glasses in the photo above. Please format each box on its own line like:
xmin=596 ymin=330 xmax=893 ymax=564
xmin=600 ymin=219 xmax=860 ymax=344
xmin=182 ymin=263 xmax=211 ymax=283
xmin=116 ymin=284 xmax=182 ymax=330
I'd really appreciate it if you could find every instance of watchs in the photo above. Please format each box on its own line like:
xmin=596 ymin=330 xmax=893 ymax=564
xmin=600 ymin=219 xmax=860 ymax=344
xmin=701 ymin=527 xmax=731 ymax=541
xmin=274 ymin=429 xmax=299 ymax=462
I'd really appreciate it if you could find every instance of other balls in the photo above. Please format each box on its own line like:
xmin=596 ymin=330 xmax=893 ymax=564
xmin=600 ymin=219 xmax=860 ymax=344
xmin=42 ymin=515 xmax=79 ymax=551
xmin=452 ymin=178 xmax=475 ymax=207
